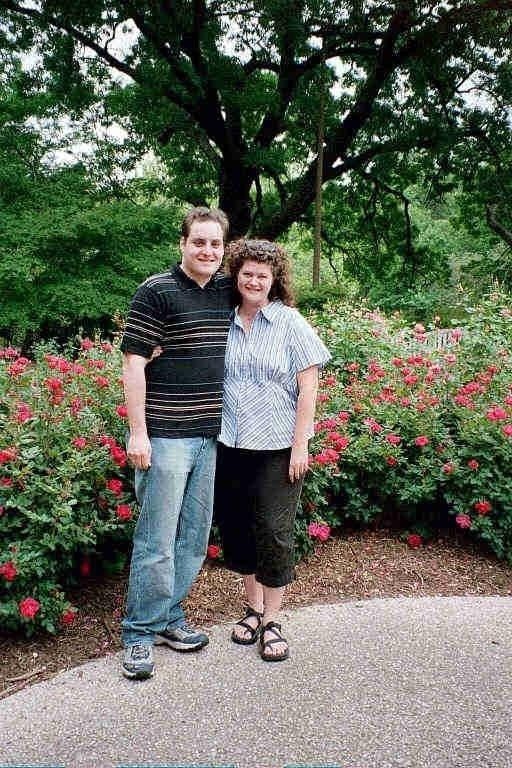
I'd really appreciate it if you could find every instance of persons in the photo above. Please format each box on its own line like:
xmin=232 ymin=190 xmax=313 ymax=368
xmin=120 ymin=205 xmax=242 ymax=682
xmin=214 ymin=239 xmax=332 ymax=662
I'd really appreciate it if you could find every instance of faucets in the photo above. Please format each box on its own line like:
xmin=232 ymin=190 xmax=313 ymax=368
xmin=231 ymin=608 xmax=290 ymax=660
xmin=121 ymin=624 xmax=210 ymax=680
